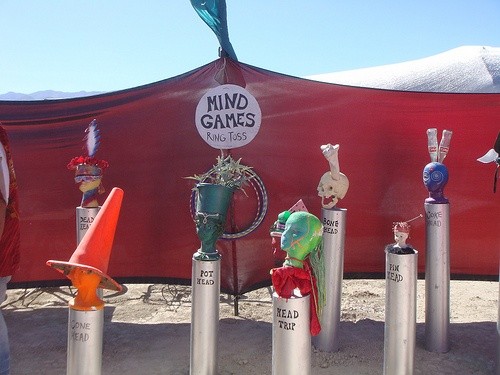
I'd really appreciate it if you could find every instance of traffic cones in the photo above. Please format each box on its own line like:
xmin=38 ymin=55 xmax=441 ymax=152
xmin=45 ymin=187 xmax=122 ymax=292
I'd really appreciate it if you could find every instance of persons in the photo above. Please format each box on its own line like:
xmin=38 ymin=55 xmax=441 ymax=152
xmin=0 ymin=123 xmax=20 ymax=375
